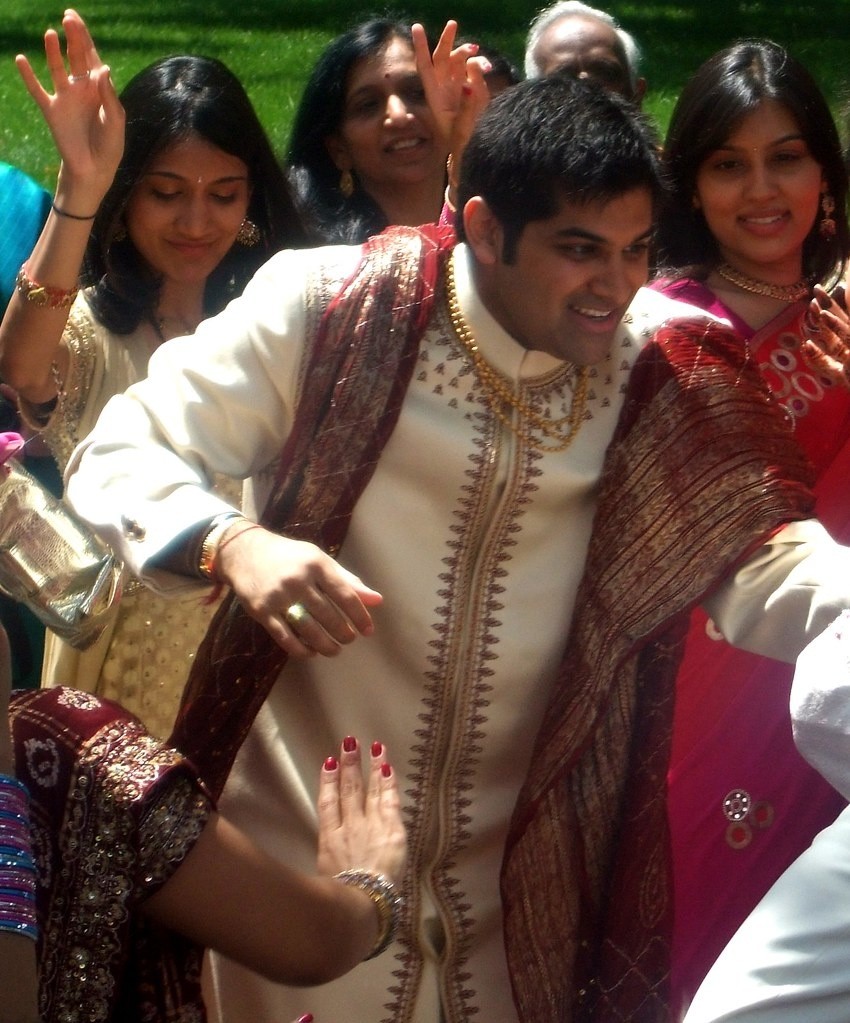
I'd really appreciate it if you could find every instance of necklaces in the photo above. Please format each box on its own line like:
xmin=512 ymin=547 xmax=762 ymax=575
xmin=150 ymin=307 xmax=167 ymax=343
xmin=715 ymin=260 xmax=814 ymax=300
xmin=446 ymin=255 xmax=588 ymax=453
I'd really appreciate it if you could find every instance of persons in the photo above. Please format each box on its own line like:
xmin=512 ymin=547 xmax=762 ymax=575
xmin=0 ymin=626 xmax=39 ymax=1023
xmin=452 ymin=35 xmax=513 ymax=98
xmin=0 ymin=8 xmax=321 ymax=740
xmin=10 ymin=684 xmax=407 ymax=1023
xmin=660 ymin=35 xmax=850 ymax=1023
xmin=523 ymin=0 xmax=647 ymax=113
xmin=284 ymin=14 xmax=493 ymax=249
xmin=63 ymin=71 xmax=850 ymax=1023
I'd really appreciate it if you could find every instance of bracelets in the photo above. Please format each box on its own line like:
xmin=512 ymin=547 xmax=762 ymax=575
xmin=0 ymin=774 xmax=37 ymax=941
xmin=445 ymin=187 xmax=457 ymax=213
xmin=51 ymin=204 xmax=95 ymax=221
xmin=447 ymin=153 xmax=461 ymax=188
xmin=17 ymin=263 xmax=78 ymax=309
xmin=332 ymin=870 xmax=402 ymax=960
xmin=183 ymin=511 xmax=254 ymax=580
